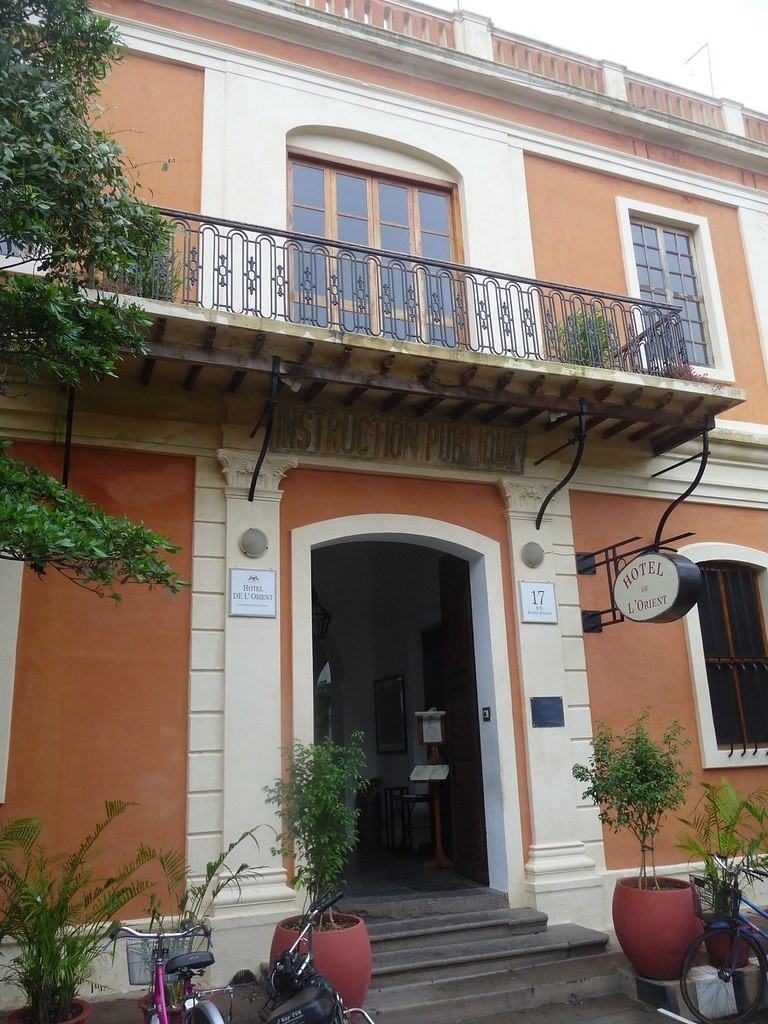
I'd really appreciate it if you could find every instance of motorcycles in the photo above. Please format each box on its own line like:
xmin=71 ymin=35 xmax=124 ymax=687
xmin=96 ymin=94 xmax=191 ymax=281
xmin=259 ymin=885 xmax=375 ymax=1024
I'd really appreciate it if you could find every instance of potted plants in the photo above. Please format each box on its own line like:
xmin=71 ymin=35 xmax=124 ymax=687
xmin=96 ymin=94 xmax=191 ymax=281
xmin=261 ymin=727 xmax=371 ymax=1024
xmin=0 ymin=799 xmax=278 ymax=1024
xmin=573 ymin=703 xmax=768 ymax=982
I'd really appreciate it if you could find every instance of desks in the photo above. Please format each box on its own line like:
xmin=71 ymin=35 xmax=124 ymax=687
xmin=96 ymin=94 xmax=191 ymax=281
xmin=393 ymin=794 xmax=436 ymax=859
xmin=384 ymin=787 xmax=411 ymax=853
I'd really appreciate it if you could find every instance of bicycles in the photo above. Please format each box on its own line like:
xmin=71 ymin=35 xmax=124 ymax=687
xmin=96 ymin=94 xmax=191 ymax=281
xmin=679 ymin=850 xmax=768 ymax=1024
xmin=103 ymin=918 xmax=235 ymax=1024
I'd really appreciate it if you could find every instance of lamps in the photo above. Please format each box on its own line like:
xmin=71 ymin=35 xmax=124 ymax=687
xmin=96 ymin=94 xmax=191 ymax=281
xmin=522 ymin=542 xmax=544 ymax=568
xmin=239 ymin=528 xmax=269 ymax=558
xmin=312 ymin=587 xmax=333 ymax=641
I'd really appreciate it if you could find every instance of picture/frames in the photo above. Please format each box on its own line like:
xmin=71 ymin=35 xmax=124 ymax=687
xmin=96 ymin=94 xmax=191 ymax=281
xmin=373 ymin=676 xmax=408 ymax=754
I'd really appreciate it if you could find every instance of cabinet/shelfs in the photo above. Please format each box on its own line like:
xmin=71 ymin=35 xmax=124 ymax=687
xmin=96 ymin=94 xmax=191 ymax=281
xmin=353 ymin=799 xmax=382 ymax=846
